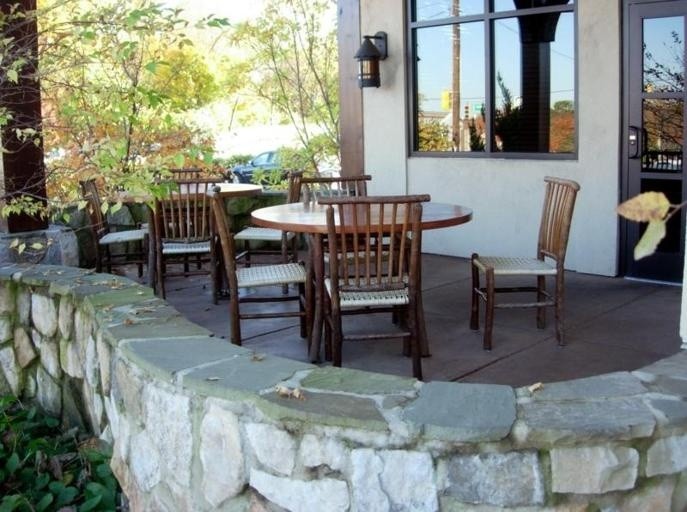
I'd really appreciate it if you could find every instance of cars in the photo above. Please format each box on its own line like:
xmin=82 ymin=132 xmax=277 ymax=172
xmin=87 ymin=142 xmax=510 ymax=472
xmin=225 ymin=150 xmax=279 ymax=183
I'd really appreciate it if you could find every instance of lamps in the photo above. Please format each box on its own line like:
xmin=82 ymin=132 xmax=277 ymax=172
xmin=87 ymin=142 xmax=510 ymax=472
xmin=353 ymin=31 xmax=387 ymax=88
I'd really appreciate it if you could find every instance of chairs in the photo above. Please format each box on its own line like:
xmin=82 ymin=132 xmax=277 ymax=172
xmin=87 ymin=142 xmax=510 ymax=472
xmin=79 ymin=168 xmax=304 ymax=306
xmin=212 ymin=187 xmax=316 ymax=358
xmin=470 ymin=176 xmax=579 ymax=350
xmin=300 ymin=174 xmax=397 ymax=325
xmin=318 ymin=194 xmax=430 ymax=380
